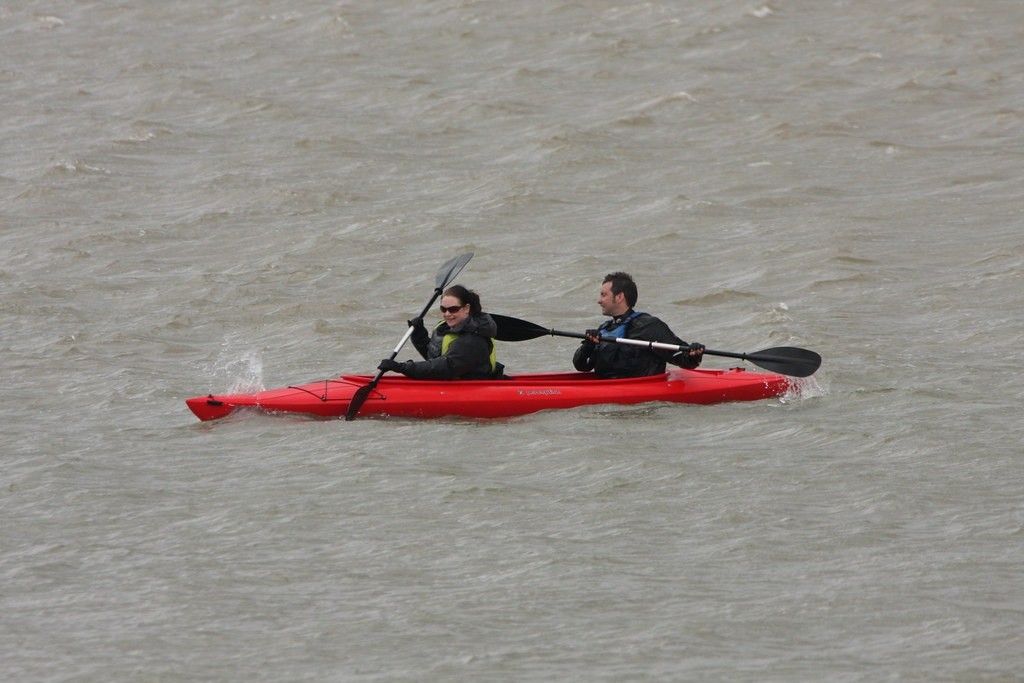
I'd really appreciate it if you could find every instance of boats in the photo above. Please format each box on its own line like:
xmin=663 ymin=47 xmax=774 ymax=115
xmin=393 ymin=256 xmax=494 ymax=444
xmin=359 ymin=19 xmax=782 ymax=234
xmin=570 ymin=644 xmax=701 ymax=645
xmin=185 ymin=370 xmax=791 ymax=421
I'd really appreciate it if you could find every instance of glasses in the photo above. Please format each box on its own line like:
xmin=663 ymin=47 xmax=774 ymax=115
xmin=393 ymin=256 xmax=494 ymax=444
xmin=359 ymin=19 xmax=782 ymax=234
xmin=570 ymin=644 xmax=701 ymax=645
xmin=440 ymin=304 xmax=465 ymax=313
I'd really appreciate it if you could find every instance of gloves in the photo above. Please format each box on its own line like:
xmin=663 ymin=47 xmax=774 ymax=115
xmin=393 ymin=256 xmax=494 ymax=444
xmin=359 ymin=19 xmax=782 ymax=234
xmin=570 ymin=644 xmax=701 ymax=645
xmin=408 ymin=316 xmax=424 ymax=333
xmin=377 ymin=359 xmax=401 ymax=373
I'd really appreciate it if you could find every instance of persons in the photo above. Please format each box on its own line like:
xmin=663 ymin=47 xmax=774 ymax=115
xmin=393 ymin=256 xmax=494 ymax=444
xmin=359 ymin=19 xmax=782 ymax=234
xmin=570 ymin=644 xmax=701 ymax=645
xmin=573 ymin=273 xmax=705 ymax=377
xmin=377 ymin=285 xmax=497 ymax=380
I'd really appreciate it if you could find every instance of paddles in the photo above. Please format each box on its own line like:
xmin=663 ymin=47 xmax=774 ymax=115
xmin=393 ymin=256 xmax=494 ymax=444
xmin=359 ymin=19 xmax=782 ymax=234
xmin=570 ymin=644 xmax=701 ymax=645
xmin=344 ymin=253 xmax=475 ymax=422
xmin=486 ymin=312 xmax=822 ymax=379
